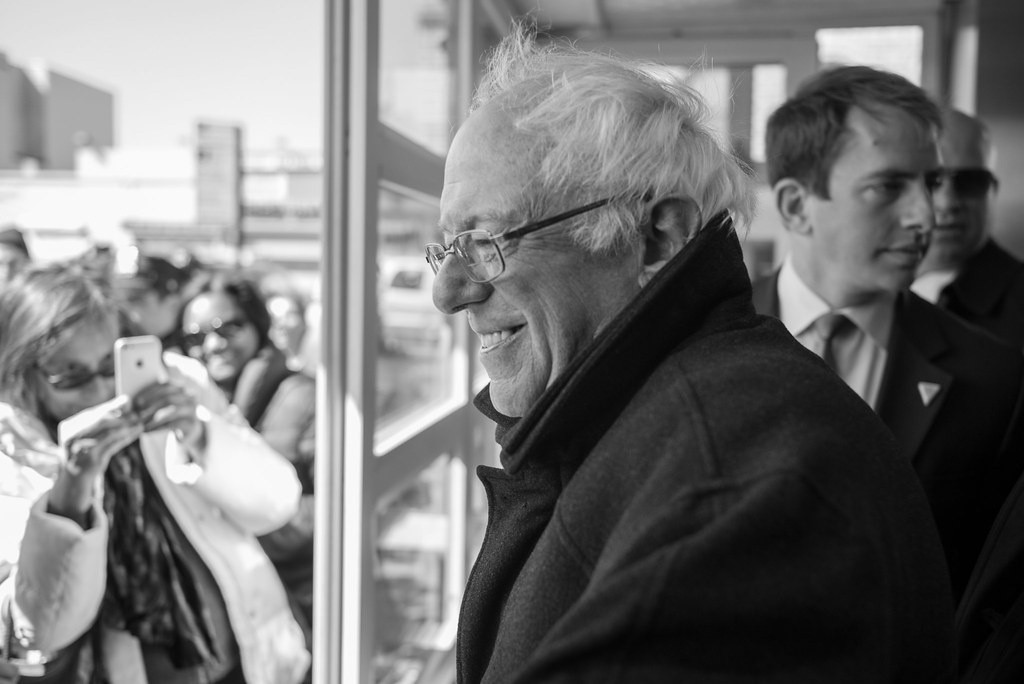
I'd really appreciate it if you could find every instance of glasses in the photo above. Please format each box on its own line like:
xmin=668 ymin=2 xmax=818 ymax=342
xmin=183 ymin=315 xmax=252 ymax=349
xmin=33 ymin=354 xmax=119 ymax=388
xmin=926 ymin=166 xmax=1000 ymax=199
xmin=425 ymin=192 xmax=654 ymax=284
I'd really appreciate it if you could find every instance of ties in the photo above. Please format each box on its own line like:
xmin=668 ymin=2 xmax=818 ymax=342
xmin=815 ymin=313 xmax=850 ymax=371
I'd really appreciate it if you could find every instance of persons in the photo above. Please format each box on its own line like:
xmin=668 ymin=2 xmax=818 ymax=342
xmin=911 ymin=109 xmax=1024 ymax=355
xmin=749 ymin=65 xmax=1023 ymax=684
xmin=431 ymin=50 xmax=965 ymax=684
xmin=0 ymin=227 xmax=317 ymax=684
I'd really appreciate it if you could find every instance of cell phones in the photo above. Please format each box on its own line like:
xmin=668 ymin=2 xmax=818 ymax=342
xmin=113 ymin=334 xmax=163 ymax=396
